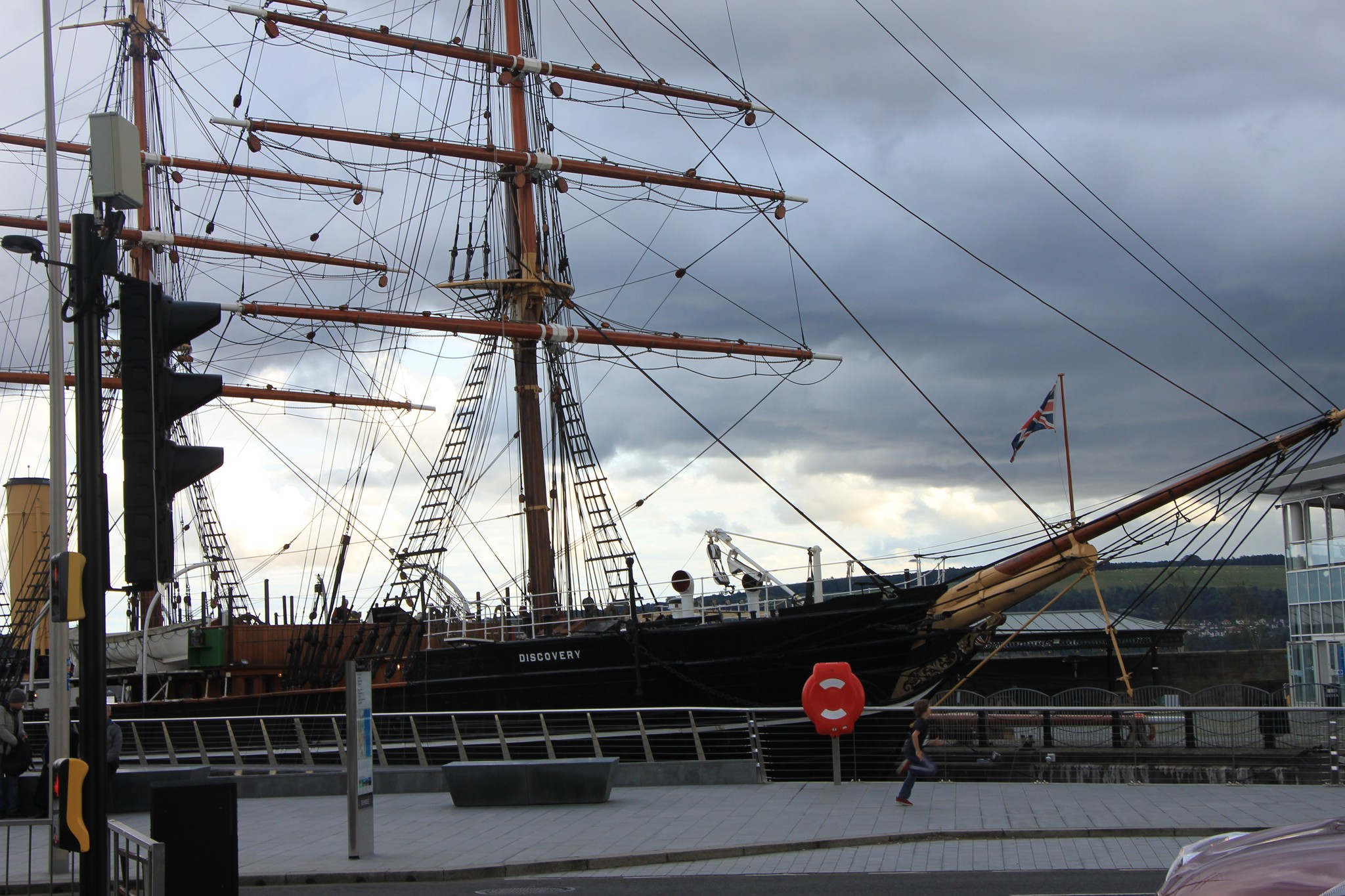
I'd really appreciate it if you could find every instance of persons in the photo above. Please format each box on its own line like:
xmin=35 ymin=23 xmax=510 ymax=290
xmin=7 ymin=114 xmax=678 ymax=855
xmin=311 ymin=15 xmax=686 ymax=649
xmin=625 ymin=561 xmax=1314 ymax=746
xmin=106 ymin=704 xmax=122 ymax=778
xmin=0 ymin=688 xmax=28 ymax=818
xmin=332 ymin=600 xmax=350 ymax=623
xmin=33 ymin=721 xmax=79 ymax=819
xmin=894 ymin=699 xmax=945 ymax=805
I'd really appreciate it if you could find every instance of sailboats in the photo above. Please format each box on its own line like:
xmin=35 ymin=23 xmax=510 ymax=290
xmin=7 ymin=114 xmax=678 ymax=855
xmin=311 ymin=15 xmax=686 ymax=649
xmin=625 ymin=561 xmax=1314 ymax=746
xmin=0 ymin=0 xmax=1345 ymax=776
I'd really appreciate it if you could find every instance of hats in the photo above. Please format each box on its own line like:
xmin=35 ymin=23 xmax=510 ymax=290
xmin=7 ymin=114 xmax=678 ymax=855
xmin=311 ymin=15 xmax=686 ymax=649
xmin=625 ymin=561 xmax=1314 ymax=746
xmin=8 ymin=688 xmax=27 ymax=703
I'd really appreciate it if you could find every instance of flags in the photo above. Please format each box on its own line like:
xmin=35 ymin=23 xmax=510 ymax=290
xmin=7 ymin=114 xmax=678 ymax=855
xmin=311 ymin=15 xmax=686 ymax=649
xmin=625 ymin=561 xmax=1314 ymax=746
xmin=1010 ymin=384 xmax=1056 ymax=464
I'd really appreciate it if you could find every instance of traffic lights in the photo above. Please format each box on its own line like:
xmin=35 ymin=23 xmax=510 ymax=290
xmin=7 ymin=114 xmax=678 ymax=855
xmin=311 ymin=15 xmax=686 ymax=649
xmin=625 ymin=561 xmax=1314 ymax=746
xmin=50 ymin=757 xmax=91 ymax=853
xmin=119 ymin=274 xmax=223 ymax=586
xmin=49 ymin=550 xmax=99 ymax=623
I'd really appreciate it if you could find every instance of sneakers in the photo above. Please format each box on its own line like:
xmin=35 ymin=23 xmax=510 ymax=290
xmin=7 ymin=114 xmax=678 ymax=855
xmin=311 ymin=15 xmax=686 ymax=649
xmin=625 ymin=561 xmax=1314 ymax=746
xmin=899 ymin=759 xmax=911 ymax=774
xmin=895 ymin=795 xmax=913 ymax=805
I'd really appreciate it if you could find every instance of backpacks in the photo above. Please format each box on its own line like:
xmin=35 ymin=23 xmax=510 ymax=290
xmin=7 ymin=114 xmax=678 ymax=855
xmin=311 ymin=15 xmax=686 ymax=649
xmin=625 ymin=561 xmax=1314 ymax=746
xmin=6 ymin=741 xmax=35 ymax=777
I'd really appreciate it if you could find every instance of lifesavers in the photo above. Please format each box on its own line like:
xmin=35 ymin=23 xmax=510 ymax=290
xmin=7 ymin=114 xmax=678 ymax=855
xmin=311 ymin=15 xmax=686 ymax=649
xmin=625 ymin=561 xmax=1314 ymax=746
xmin=1128 ymin=712 xmax=1156 ymax=742
